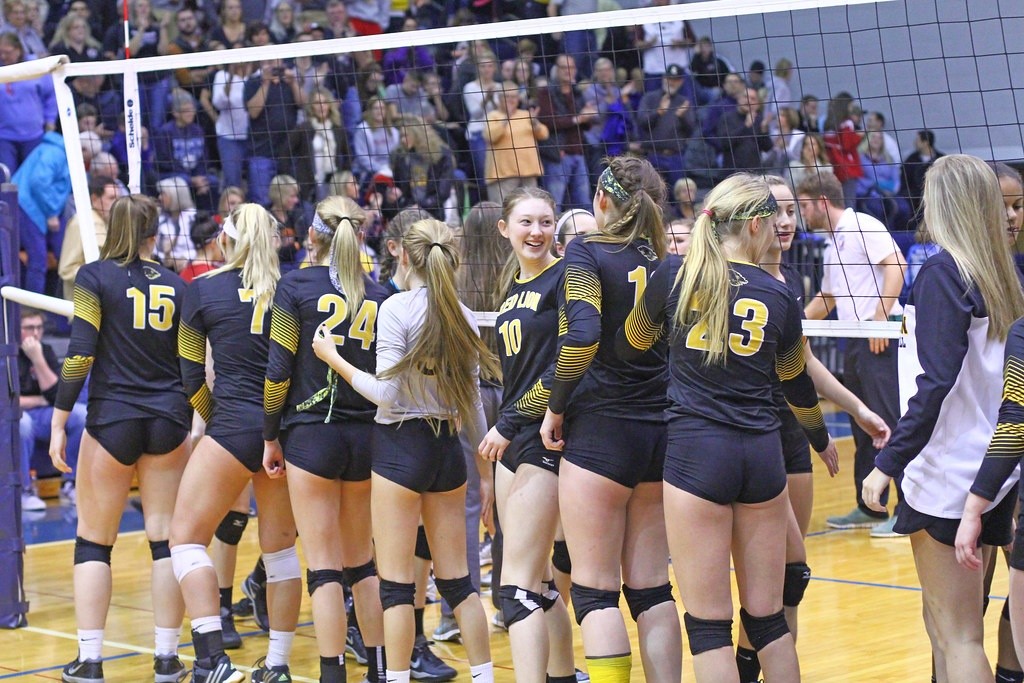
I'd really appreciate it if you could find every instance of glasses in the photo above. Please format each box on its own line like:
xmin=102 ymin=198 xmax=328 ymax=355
xmin=21 ymin=325 xmax=45 ymax=332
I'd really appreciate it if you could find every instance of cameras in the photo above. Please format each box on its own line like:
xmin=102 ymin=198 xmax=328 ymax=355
xmin=272 ymin=66 xmax=284 ymax=76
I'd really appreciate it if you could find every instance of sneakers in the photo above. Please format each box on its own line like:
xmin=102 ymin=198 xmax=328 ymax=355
xmin=63 ymin=657 xmax=105 ymax=683
xmin=410 ymin=635 xmax=457 ymax=681
xmin=190 ymin=654 xmax=245 ymax=683
xmin=243 ymin=573 xmax=269 ymax=631
xmin=345 ymin=626 xmax=368 ymax=665
xmin=154 ymin=654 xmax=185 ymax=682
xmin=251 ymin=656 xmax=293 ymax=683
xmin=220 ymin=606 xmax=241 ymax=648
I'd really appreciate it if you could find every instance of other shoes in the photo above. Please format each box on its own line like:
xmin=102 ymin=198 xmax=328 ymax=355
xmin=493 ymin=608 xmax=508 ymax=630
xmin=870 ymin=515 xmax=906 ymax=538
xmin=433 ymin=620 xmax=460 ymax=641
xmin=825 ymin=507 xmax=889 ymax=529
xmin=480 ymin=536 xmax=493 ymax=566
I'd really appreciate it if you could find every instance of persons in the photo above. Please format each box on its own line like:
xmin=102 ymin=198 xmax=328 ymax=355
xmin=483 ymin=81 xmax=548 ymax=203
xmin=796 ymin=172 xmax=912 ymax=539
xmin=540 ymin=152 xmax=683 ymax=683
xmin=0 ymin=0 xmax=1024 ymax=683
xmin=311 ymin=219 xmax=498 ymax=683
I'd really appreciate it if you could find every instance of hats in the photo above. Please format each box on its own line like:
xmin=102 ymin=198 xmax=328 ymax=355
xmin=666 ymin=65 xmax=685 ymax=79
